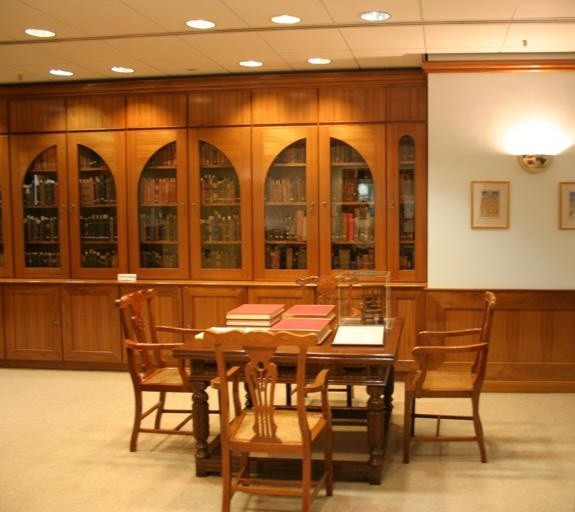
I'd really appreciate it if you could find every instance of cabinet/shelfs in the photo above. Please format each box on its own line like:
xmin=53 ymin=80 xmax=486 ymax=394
xmin=388 ymin=288 xmax=427 ymax=372
xmin=8 ymin=130 xmax=128 ymax=280
xmin=382 ymin=120 xmax=426 ymax=283
xmin=1 ymin=133 xmax=15 ymax=279
xmin=246 ymin=286 xmax=385 ymax=319
xmin=126 ymin=125 xmax=253 ymax=282
xmin=2 ymin=282 xmax=122 ymax=366
xmin=251 ymin=121 xmax=387 ymax=281
xmin=119 ymin=282 xmax=248 ymax=367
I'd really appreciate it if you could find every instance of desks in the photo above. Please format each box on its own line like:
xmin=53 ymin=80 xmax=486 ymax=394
xmin=172 ymin=315 xmax=405 ymax=484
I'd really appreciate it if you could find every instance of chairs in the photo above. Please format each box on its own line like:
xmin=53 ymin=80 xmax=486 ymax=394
xmin=404 ymin=291 xmax=498 ymax=463
xmin=114 ymin=288 xmax=221 ymax=451
xmin=203 ymin=329 xmax=334 ymax=512
xmin=286 ymin=275 xmax=359 ymax=406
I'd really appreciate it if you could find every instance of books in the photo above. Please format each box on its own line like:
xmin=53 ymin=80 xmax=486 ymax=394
xmin=264 ymin=138 xmax=307 ymax=270
xmin=22 ymin=145 xmax=60 ymax=267
xmin=194 ymin=303 xmax=385 ymax=346
xmin=399 ymin=134 xmax=417 ymax=271
xmin=78 ymin=144 xmax=119 ymax=268
xmin=198 ymin=140 xmax=241 ymax=267
xmin=330 ymin=136 xmax=375 ymax=271
xmin=138 ymin=141 xmax=178 ymax=267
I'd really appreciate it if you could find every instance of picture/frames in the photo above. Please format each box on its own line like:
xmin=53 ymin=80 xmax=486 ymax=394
xmin=560 ymin=181 xmax=575 ymax=230
xmin=471 ymin=180 xmax=509 ymax=230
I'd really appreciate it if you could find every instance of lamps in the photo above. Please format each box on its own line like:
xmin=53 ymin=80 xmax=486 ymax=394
xmin=519 ymin=154 xmax=553 ymax=174
